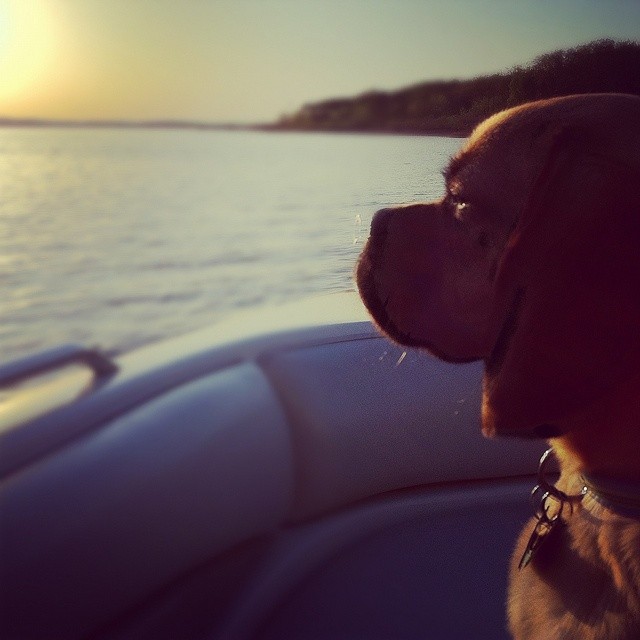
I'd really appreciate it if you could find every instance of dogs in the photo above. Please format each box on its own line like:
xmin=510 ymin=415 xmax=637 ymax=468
xmin=353 ymin=88 xmax=640 ymax=640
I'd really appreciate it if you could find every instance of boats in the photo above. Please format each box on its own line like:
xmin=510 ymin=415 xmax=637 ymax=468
xmin=0 ymin=293 xmax=561 ymax=639
